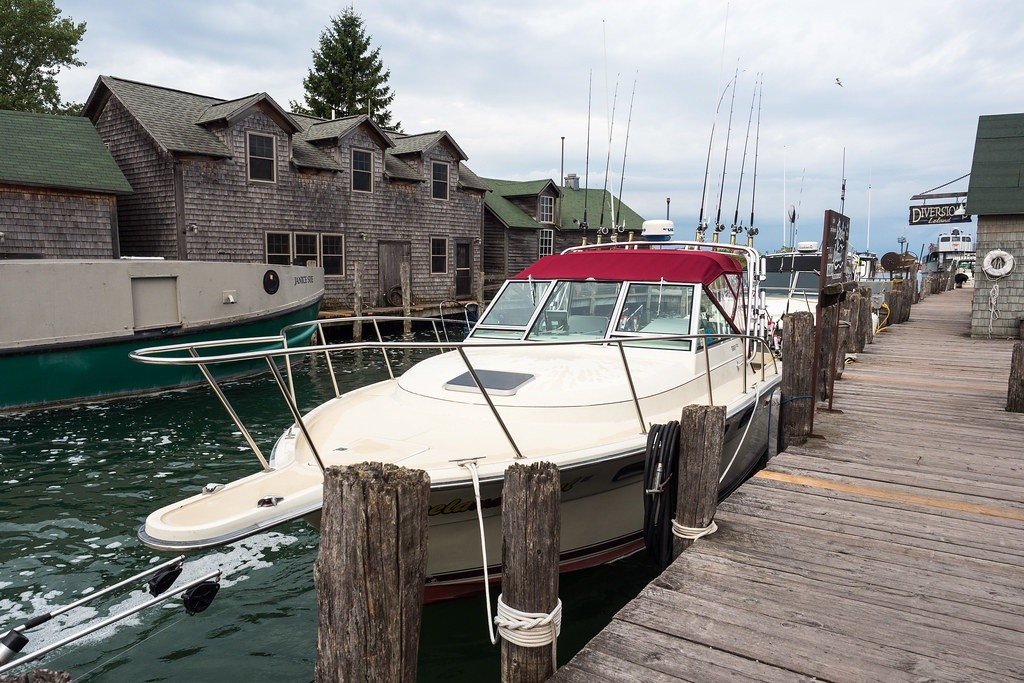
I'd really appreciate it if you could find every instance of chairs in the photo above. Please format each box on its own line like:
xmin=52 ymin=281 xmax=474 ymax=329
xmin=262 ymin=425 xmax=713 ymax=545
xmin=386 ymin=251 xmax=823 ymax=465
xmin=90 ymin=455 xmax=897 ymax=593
xmin=567 ymin=312 xmax=609 ymax=334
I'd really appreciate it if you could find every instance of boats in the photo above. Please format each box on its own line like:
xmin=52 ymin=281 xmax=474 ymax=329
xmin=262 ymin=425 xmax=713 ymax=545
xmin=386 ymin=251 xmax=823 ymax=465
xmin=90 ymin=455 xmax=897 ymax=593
xmin=0 ymin=256 xmax=326 ymax=415
xmin=128 ymin=53 xmax=880 ymax=608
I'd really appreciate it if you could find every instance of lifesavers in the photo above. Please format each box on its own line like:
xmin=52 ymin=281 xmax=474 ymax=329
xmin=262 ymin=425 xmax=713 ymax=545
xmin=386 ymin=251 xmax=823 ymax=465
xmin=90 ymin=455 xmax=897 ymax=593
xmin=982 ymin=249 xmax=1013 ymax=277
